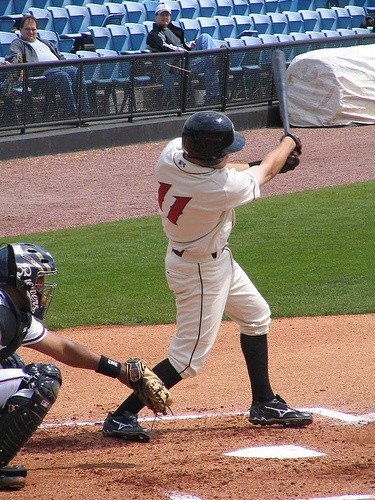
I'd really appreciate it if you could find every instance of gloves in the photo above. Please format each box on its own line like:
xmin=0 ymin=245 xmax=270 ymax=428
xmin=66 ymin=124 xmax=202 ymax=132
xmin=280 ymin=155 xmax=299 ymax=172
xmin=280 ymin=133 xmax=302 ymax=150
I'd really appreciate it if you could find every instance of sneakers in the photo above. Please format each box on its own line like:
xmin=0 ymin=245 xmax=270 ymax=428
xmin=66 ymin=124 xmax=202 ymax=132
xmin=248 ymin=393 xmax=313 ymax=428
xmin=0 ymin=464 xmax=27 ymax=488
xmin=102 ymin=410 xmax=150 ymax=442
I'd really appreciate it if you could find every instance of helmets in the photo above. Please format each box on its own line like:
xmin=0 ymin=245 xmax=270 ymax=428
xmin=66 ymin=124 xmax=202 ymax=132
xmin=0 ymin=242 xmax=58 ymax=321
xmin=181 ymin=111 xmax=245 ymax=165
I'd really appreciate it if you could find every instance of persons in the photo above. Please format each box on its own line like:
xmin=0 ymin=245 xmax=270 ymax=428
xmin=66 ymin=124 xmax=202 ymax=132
xmin=146 ymin=4 xmax=230 ymax=106
xmin=0 ymin=16 xmax=91 ymax=125
xmin=0 ymin=243 xmax=175 ymax=492
xmin=102 ymin=111 xmax=313 ymax=441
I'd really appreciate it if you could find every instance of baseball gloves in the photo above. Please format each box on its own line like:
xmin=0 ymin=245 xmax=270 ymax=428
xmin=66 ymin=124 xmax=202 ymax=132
xmin=127 ymin=355 xmax=176 ymax=414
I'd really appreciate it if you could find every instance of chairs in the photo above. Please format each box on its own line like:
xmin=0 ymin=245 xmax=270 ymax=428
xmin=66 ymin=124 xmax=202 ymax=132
xmin=0 ymin=0 xmax=375 ymax=125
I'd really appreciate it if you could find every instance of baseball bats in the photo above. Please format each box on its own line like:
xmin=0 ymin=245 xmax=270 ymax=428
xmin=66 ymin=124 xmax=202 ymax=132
xmin=271 ymin=48 xmax=296 ymax=165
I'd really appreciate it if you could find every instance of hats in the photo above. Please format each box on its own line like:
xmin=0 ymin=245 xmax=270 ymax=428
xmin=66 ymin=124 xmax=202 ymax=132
xmin=155 ymin=4 xmax=171 ymax=14
